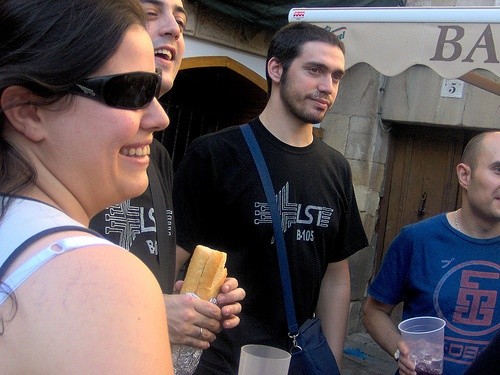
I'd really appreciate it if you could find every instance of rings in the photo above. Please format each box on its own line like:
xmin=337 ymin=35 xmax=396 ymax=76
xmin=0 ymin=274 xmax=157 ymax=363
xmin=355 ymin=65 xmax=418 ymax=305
xmin=197 ymin=327 xmax=203 ymax=338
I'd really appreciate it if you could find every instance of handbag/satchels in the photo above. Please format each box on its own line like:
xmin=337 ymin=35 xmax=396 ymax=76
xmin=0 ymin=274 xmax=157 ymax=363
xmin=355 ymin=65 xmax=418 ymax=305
xmin=288 ymin=318 xmax=341 ymax=375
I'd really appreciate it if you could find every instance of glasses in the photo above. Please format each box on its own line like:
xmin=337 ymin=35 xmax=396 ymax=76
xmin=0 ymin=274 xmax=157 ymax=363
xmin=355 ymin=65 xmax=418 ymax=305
xmin=33 ymin=72 xmax=161 ymax=109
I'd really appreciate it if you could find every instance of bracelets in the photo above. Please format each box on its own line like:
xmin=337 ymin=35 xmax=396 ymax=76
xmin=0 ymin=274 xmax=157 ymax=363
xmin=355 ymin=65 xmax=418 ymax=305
xmin=393 ymin=349 xmax=400 ymax=362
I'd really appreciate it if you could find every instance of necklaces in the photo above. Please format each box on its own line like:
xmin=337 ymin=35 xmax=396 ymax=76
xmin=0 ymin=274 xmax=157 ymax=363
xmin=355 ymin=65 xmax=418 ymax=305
xmin=454 ymin=207 xmax=462 ymax=231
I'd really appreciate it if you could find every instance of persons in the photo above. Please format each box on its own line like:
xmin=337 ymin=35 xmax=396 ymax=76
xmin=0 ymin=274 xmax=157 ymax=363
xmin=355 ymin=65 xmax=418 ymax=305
xmin=363 ymin=130 xmax=500 ymax=375
xmin=88 ymin=0 xmax=246 ymax=375
xmin=172 ymin=21 xmax=370 ymax=375
xmin=0 ymin=0 xmax=175 ymax=375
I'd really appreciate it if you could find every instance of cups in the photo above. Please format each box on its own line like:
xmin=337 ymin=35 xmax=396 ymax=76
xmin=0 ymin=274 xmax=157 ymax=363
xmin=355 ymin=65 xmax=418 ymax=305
xmin=398 ymin=316 xmax=446 ymax=375
xmin=236 ymin=344 xmax=292 ymax=375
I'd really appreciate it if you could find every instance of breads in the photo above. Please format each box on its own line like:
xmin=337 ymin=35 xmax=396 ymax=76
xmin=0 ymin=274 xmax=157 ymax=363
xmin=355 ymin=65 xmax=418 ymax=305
xmin=180 ymin=244 xmax=227 ymax=301
